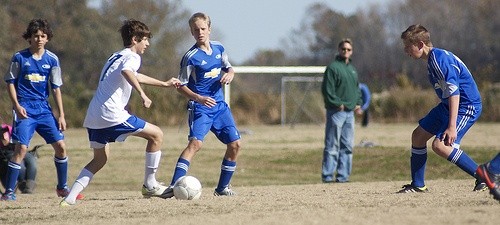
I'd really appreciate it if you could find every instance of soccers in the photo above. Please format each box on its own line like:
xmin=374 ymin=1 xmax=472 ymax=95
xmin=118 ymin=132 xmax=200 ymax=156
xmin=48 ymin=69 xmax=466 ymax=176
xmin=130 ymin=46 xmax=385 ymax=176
xmin=173 ymin=175 xmax=201 ymax=201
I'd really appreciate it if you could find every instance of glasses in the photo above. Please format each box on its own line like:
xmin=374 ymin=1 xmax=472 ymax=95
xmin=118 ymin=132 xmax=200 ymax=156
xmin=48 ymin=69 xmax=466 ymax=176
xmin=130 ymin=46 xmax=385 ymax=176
xmin=340 ymin=48 xmax=352 ymax=51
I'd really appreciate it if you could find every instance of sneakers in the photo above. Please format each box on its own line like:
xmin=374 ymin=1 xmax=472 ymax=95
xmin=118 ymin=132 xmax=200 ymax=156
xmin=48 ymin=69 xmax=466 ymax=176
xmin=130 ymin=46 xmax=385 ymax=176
xmin=214 ymin=189 xmax=236 ymax=196
xmin=474 ymin=161 xmax=500 ymax=202
xmin=473 ymin=176 xmax=488 ymax=193
xmin=56 ymin=187 xmax=84 ymax=200
xmin=1 ymin=188 xmax=16 ymax=200
xmin=142 ymin=182 xmax=169 ymax=199
xmin=160 ymin=185 xmax=174 ymax=199
xmin=60 ymin=198 xmax=75 ymax=207
xmin=396 ymin=182 xmax=428 ymax=194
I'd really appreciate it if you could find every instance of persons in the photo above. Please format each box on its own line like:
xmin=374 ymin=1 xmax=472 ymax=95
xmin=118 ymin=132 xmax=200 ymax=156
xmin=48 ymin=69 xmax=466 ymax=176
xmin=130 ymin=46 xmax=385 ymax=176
xmin=478 ymin=152 xmax=500 ymax=201
xmin=359 ymin=83 xmax=371 ymax=127
xmin=397 ymin=24 xmax=488 ymax=194
xmin=0 ymin=125 xmax=37 ymax=194
xmin=0 ymin=18 xmax=71 ymax=200
xmin=321 ymin=39 xmax=361 ymax=183
xmin=169 ymin=12 xmax=239 ymax=197
xmin=58 ymin=19 xmax=182 ymax=207
